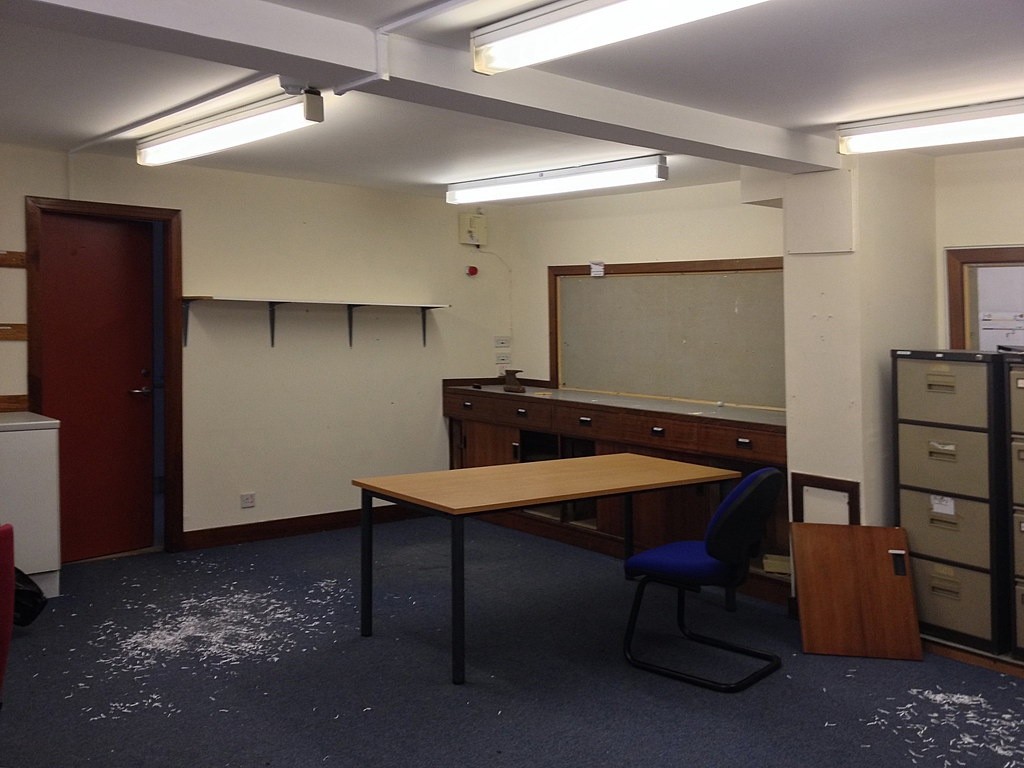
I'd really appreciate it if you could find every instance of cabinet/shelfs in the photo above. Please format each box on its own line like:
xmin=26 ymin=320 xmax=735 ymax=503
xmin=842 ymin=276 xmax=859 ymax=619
xmin=889 ymin=348 xmax=1024 ymax=657
xmin=445 ymin=384 xmax=787 ymax=592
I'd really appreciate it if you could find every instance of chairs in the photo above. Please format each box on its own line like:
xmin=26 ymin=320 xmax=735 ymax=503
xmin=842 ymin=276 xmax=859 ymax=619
xmin=623 ymin=468 xmax=782 ymax=692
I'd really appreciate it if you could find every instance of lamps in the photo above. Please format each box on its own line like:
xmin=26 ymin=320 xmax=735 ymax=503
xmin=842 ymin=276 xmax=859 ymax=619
xmin=470 ymin=0 xmax=771 ymax=78
xmin=136 ymin=88 xmax=325 ymax=167
xmin=836 ymin=97 xmax=1024 ymax=156
xmin=445 ymin=154 xmax=669 ymax=206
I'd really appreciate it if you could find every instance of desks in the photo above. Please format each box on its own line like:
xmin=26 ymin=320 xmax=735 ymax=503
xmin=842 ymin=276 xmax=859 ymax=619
xmin=351 ymin=453 xmax=741 ymax=684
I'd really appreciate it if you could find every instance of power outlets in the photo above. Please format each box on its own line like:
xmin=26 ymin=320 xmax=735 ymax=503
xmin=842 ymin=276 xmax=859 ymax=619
xmin=241 ymin=492 xmax=256 ymax=508
